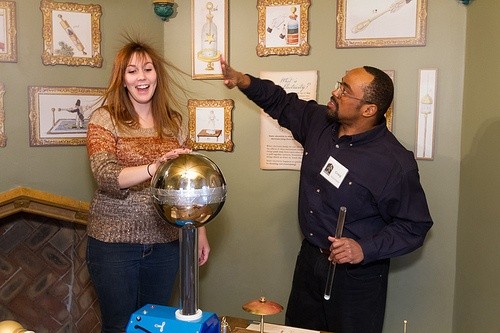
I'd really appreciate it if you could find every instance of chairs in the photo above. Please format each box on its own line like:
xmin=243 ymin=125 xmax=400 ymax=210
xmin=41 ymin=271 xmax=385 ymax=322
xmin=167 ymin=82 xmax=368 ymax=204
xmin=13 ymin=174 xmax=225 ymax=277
xmin=0 ymin=183 xmax=103 ymax=333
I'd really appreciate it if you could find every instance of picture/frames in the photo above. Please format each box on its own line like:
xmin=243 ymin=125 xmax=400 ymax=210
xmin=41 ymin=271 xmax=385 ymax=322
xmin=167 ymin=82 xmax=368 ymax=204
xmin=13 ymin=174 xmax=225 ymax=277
xmin=187 ymin=99 xmax=235 ymax=152
xmin=40 ymin=0 xmax=104 ymax=68
xmin=191 ymin=0 xmax=228 ymax=79
xmin=415 ymin=67 xmax=438 ymax=162
xmin=336 ymin=0 xmax=428 ymax=49
xmin=0 ymin=0 xmax=18 ymax=62
xmin=256 ymin=0 xmax=312 ymax=57
xmin=347 ymin=70 xmax=396 ymax=134
xmin=27 ymin=86 xmax=109 ymax=148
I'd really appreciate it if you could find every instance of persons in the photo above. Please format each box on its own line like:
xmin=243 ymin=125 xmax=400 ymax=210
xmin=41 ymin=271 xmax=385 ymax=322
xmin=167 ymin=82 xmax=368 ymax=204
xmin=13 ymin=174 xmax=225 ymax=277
xmin=220 ymin=54 xmax=434 ymax=333
xmin=65 ymin=100 xmax=83 ymax=129
xmin=86 ymin=31 xmax=220 ymax=333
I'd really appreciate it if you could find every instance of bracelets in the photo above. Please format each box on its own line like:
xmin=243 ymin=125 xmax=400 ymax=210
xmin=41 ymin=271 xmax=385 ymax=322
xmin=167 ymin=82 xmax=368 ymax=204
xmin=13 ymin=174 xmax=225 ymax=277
xmin=146 ymin=163 xmax=153 ymax=176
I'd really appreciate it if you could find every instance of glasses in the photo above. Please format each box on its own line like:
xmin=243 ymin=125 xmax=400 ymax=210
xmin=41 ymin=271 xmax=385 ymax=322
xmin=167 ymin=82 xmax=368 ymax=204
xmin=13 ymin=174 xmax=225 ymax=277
xmin=335 ymin=81 xmax=379 ymax=111
